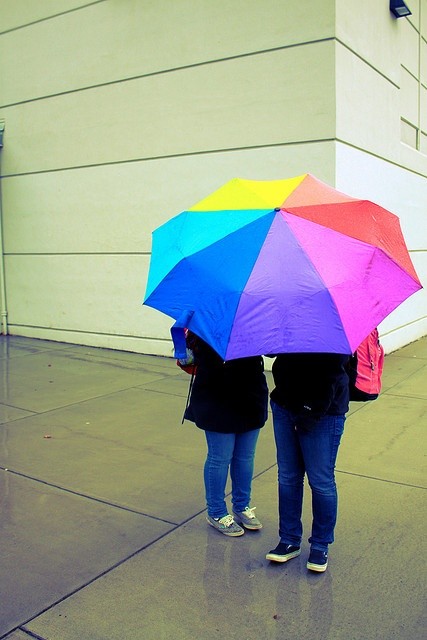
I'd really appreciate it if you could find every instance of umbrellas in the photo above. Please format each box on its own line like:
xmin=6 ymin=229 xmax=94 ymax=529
xmin=141 ymin=173 xmax=424 ymax=365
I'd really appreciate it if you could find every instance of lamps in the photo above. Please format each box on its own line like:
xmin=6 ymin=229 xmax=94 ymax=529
xmin=389 ymin=0 xmax=412 ymax=18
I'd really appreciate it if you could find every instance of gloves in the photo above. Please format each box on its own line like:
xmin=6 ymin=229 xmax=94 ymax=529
xmin=291 ymin=403 xmax=317 ymax=434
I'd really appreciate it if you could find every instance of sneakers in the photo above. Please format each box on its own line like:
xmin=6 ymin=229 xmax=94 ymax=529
xmin=231 ymin=507 xmax=262 ymax=529
xmin=206 ymin=514 xmax=244 ymax=537
xmin=307 ymin=550 xmax=328 ymax=572
xmin=266 ymin=543 xmax=301 ymax=562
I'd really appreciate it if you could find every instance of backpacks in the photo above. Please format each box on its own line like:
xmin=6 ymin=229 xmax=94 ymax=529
xmin=350 ymin=328 xmax=384 ymax=401
xmin=177 ymin=328 xmax=197 ymax=375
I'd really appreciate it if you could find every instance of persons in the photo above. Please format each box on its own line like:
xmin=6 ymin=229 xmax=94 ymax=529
xmin=265 ymin=353 xmax=350 ymax=573
xmin=184 ymin=329 xmax=269 ymax=537
